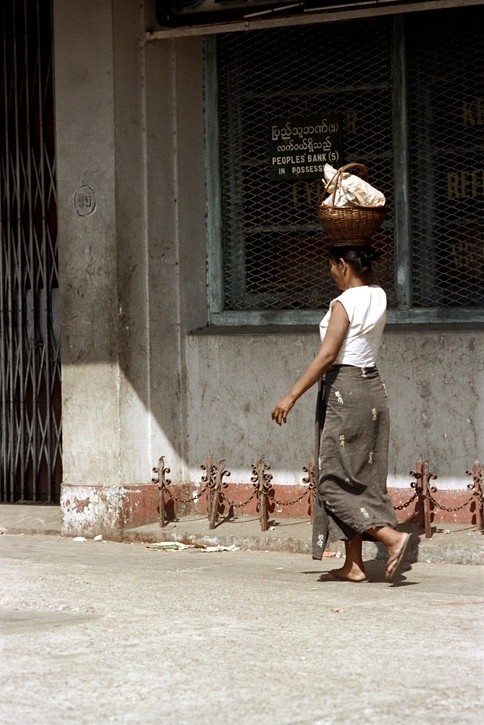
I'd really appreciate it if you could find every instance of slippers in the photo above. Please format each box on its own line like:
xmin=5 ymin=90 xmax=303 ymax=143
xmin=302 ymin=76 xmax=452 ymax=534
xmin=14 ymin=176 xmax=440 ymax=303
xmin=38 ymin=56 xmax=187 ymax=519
xmin=320 ymin=569 xmax=372 ymax=583
xmin=385 ymin=533 xmax=419 ymax=582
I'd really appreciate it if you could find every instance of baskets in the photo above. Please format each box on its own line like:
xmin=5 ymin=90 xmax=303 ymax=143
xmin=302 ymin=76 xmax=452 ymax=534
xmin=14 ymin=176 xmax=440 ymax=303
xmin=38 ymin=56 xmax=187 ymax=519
xmin=315 ymin=162 xmax=386 ymax=247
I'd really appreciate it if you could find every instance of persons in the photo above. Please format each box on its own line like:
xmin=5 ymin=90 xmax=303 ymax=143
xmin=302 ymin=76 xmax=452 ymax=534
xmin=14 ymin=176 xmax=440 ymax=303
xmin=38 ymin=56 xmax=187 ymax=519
xmin=271 ymin=244 xmax=418 ymax=586
